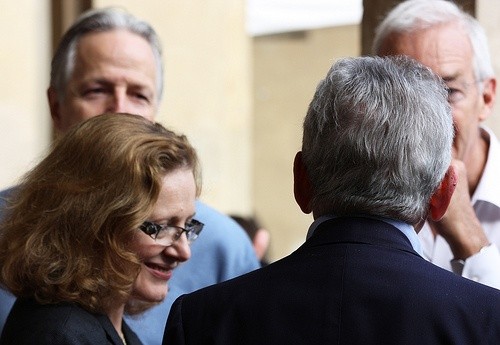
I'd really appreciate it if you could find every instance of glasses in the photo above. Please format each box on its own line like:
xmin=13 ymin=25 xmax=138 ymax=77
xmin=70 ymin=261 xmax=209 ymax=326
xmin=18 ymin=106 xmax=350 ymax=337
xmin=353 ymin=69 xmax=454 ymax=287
xmin=137 ymin=219 xmax=205 ymax=247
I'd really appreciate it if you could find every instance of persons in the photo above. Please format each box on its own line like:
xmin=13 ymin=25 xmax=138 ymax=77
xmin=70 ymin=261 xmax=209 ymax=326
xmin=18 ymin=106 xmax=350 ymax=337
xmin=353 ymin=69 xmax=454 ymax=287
xmin=0 ymin=113 xmax=205 ymax=345
xmin=1 ymin=7 xmax=270 ymax=345
xmin=371 ymin=0 xmax=500 ymax=291
xmin=161 ymin=57 xmax=500 ymax=345
